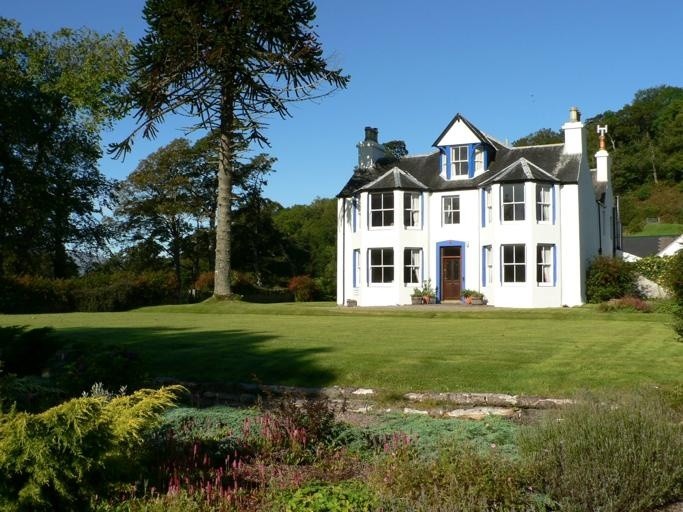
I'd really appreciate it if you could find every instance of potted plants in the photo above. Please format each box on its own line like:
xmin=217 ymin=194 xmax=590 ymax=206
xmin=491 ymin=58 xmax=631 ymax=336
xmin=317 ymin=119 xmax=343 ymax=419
xmin=410 ymin=280 xmax=433 ymax=304
xmin=461 ymin=288 xmax=484 ymax=305
